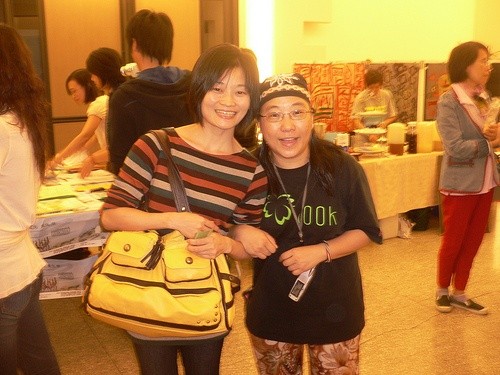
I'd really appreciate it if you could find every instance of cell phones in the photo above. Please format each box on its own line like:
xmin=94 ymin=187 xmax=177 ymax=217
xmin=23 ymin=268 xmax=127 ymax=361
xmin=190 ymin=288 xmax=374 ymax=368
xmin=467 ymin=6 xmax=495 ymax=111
xmin=287 ymin=267 xmax=316 ymax=302
xmin=193 ymin=228 xmax=213 ymax=239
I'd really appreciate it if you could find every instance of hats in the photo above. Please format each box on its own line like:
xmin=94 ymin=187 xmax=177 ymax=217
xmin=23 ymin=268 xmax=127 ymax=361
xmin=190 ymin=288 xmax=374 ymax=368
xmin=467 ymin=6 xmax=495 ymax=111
xmin=259 ymin=73 xmax=311 ymax=105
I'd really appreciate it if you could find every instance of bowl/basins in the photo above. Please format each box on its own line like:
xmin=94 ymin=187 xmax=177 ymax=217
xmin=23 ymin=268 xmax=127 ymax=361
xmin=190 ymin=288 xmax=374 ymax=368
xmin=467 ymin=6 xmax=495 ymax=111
xmin=352 ymin=129 xmax=387 ymax=144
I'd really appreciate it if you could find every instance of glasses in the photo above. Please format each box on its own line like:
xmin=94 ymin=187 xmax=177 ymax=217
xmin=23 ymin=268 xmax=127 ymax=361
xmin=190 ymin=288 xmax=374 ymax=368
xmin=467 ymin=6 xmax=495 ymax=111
xmin=259 ymin=108 xmax=313 ymax=122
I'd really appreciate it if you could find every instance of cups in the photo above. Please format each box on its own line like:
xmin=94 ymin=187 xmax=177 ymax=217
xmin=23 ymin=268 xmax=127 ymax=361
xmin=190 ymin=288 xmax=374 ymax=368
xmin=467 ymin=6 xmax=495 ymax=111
xmin=333 ymin=133 xmax=350 ymax=148
xmin=416 ymin=122 xmax=432 ymax=153
xmin=387 ymin=123 xmax=404 ymax=155
xmin=121 ymin=62 xmax=141 ymax=78
xmin=406 ymin=122 xmax=418 ymax=154
xmin=314 ymin=123 xmax=327 ymax=138
xmin=432 ymin=121 xmax=443 ymax=151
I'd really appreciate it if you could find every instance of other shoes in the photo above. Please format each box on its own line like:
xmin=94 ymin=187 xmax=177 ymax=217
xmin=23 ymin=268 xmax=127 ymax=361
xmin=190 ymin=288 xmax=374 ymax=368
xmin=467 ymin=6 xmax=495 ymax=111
xmin=434 ymin=293 xmax=451 ymax=312
xmin=449 ymin=295 xmax=488 ymax=315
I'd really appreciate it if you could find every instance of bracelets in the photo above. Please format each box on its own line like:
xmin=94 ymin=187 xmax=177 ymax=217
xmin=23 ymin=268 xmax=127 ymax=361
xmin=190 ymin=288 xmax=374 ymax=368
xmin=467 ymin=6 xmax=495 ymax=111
xmin=321 ymin=239 xmax=331 ymax=262
xmin=89 ymin=153 xmax=96 ymax=165
xmin=56 ymin=152 xmax=63 ymax=163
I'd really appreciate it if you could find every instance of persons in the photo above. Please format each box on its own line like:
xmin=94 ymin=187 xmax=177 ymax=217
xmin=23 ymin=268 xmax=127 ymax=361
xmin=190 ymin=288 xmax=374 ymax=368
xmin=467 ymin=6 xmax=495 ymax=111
xmin=227 ymin=73 xmax=382 ymax=375
xmin=45 ymin=67 xmax=109 ymax=174
xmin=352 ymin=73 xmax=398 ymax=142
xmin=435 ymin=42 xmax=500 ymax=315
xmin=106 ymin=9 xmax=195 ymax=175
xmin=99 ymin=44 xmax=268 ymax=375
xmin=0 ymin=23 xmax=62 ymax=375
xmin=79 ymin=47 xmax=135 ymax=179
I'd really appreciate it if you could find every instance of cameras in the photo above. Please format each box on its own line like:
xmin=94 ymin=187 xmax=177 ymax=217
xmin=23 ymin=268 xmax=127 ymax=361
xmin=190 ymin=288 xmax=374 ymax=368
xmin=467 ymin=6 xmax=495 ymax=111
xmin=119 ymin=63 xmax=140 ymax=78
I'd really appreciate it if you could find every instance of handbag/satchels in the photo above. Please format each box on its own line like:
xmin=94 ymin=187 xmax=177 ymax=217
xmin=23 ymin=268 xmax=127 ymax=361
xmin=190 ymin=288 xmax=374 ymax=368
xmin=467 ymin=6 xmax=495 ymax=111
xmin=82 ymin=129 xmax=240 ymax=342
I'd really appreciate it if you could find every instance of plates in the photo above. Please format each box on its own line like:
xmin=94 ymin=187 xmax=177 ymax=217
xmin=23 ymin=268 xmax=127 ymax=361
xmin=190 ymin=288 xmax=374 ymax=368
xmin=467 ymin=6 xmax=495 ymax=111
xmin=354 ymin=145 xmax=386 ymax=157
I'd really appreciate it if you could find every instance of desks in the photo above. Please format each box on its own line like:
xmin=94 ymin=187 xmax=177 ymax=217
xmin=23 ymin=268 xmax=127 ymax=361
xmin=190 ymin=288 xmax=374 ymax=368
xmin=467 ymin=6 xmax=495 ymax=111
xmin=356 ymin=151 xmax=442 ymax=219
